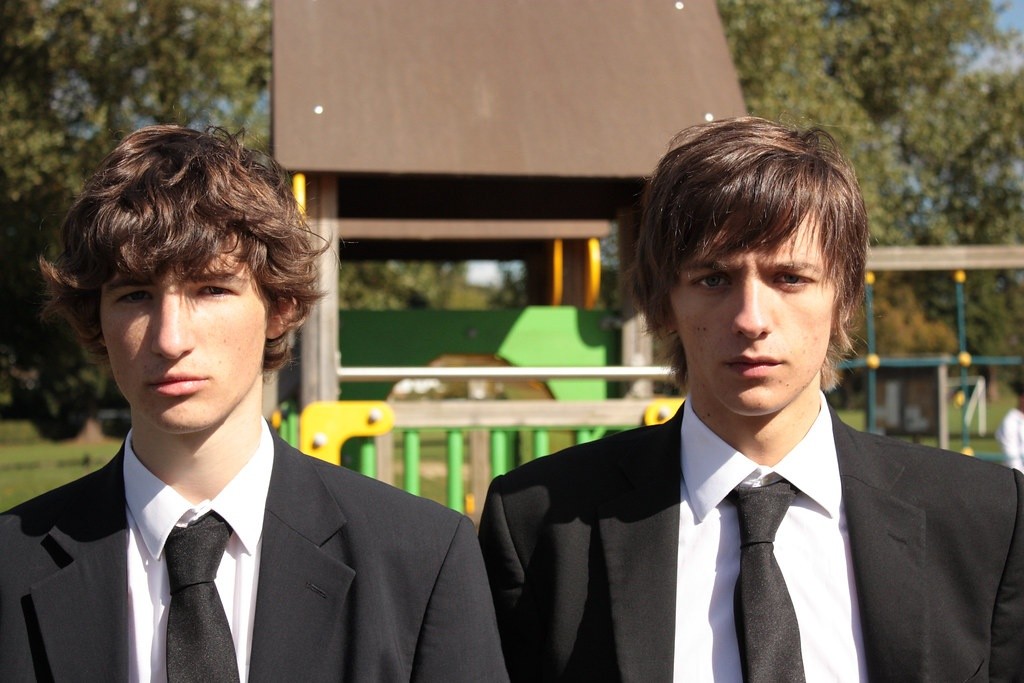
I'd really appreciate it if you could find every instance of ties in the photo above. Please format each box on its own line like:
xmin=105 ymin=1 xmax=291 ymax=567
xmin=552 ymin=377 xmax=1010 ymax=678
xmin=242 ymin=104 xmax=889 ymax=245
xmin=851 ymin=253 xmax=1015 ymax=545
xmin=724 ymin=475 xmax=806 ymax=683
xmin=164 ymin=508 xmax=241 ymax=683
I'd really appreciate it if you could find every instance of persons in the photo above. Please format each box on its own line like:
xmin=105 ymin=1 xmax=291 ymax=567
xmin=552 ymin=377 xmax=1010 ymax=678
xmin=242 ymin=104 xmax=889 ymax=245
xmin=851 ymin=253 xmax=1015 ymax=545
xmin=479 ymin=115 xmax=1024 ymax=682
xmin=995 ymin=379 xmax=1024 ymax=470
xmin=0 ymin=126 xmax=509 ymax=682
